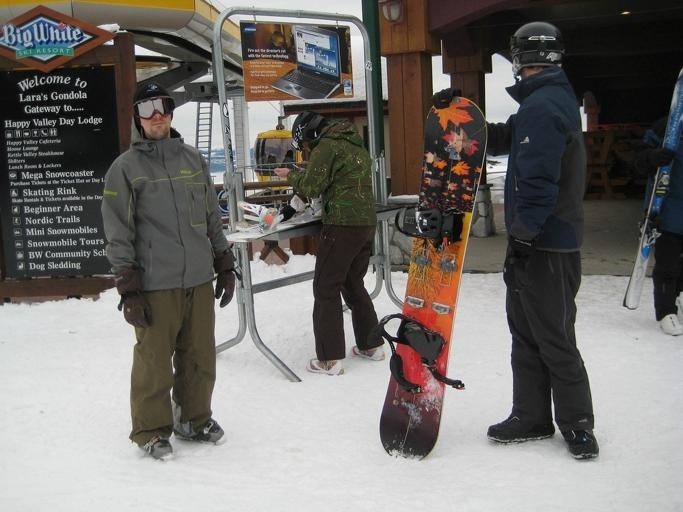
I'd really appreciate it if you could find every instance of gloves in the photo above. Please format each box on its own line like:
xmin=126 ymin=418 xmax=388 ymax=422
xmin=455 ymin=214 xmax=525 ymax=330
xmin=430 ymin=85 xmax=462 ymax=110
xmin=209 ymin=249 xmax=240 ymax=308
xmin=501 ymin=238 xmax=534 ymax=296
xmin=278 ymin=205 xmax=298 ymax=222
xmin=647 ymin=146 xmax=677 ymax=168
xmin=116 ymin=271 xmax=153 ymax=329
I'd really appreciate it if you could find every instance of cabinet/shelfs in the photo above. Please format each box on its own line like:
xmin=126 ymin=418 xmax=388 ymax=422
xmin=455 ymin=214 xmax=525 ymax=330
xmin=214 ymin=7 xmax=404 ymax=382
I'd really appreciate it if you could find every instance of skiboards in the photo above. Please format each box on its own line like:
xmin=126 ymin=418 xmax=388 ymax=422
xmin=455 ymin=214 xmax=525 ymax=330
xmin=623 ymin=68 xmax=683 ymax=310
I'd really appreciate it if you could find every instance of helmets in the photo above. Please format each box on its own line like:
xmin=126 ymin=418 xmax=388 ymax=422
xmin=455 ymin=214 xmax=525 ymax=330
xmin=288 ymin=110 xmax=325 ymax=147
xmin=132 ymin=81 xmax=174 ymax=106
xmin=507 ymin=20 xmax=568 ymax=78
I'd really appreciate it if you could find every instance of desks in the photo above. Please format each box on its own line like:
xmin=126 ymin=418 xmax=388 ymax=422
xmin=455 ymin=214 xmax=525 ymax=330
xmin=243 ymin=59 xmax=352 ymax=100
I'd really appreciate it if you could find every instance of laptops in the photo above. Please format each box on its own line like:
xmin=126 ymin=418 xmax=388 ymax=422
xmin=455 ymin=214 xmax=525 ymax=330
xmin=272 ymin=24 xmax=342 ymax=99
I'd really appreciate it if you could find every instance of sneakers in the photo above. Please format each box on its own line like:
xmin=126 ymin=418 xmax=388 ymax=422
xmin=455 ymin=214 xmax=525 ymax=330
xmin=565 ymin=422 xmax=601 ymax=461
xmin=306 ymin=357 xmax=343 ymax=377
xmin=133 ymin=434 xmax=175 ymax=462
xmin=353 ymin=342 xmax=386 ymax=363
xmin=485 ymin=412 xmax=557 ymax=444
xmin=657 ymin=314 xmax=683 ymax=337
xmin=173 ymin=415 xmax=228 ymax=447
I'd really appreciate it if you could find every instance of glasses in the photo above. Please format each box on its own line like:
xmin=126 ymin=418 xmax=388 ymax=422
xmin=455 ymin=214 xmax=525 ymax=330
xmin=136 ymin=98 xmax=176 ymax=120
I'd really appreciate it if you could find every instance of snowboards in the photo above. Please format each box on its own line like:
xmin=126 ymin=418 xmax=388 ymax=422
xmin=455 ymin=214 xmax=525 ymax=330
xmin=376 ymin=96 xmax=488 ymax=460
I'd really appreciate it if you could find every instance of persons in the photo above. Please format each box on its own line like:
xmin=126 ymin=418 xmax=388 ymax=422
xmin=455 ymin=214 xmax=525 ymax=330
xmin=99 ymin=80 xmax=240 ymax=461
xmin=273 ymin=109 xmax=386 ymax=376
xmin=433 ymin=19 xmax=601 ymax=461
xmin=629 ymin=108 xmax=683 ymax=335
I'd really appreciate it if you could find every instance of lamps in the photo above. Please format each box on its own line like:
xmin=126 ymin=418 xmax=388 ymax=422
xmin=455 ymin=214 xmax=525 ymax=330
xmin=378 ymin=0 xmax=407 ymax=25
